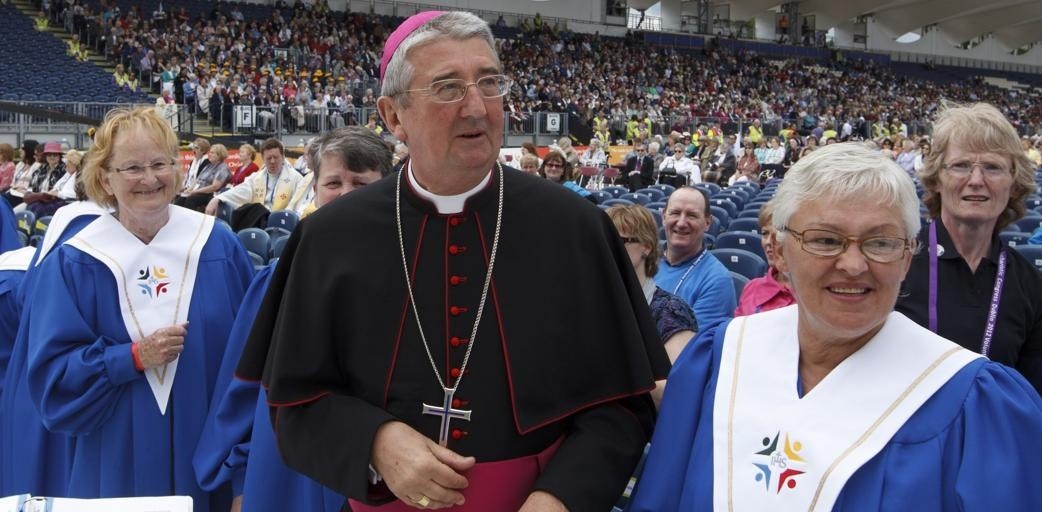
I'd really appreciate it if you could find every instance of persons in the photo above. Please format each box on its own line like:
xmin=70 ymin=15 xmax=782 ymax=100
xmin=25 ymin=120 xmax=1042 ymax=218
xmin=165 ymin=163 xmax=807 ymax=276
xmin=629 ymin=140 xmax=1042 ymax=511
xmin=485 ymin=10 xmax=1041 ymax=412
xmin=1 ymin=1 xmax=408 ymax=512
xmin=236 ymin=10 xmax=671 ymax=512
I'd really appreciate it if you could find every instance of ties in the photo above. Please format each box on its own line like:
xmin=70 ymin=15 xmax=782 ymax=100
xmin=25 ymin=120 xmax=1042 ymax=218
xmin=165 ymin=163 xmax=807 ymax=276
xmin=637 ymin=158 xmax=641 ymax=170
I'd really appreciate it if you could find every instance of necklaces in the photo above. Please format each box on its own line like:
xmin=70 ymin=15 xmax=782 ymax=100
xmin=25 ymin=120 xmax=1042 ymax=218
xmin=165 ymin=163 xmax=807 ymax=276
xmin=397 ymin=160 xmax=503 ymax=447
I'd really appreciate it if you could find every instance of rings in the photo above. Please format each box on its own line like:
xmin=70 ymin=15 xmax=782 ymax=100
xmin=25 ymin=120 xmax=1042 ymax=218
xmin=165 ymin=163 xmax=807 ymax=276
xmin=418 ymin=496 xmax=430 ymax=507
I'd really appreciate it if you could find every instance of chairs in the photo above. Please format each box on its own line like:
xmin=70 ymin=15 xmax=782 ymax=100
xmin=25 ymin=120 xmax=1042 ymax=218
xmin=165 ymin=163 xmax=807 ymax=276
xmin=17 ymin=209 xmax=52 ymax=251
xmin=217 ymin=200 xmax=299 ymax=271
xmin=0 ymin=1 xmax=157 ymax=120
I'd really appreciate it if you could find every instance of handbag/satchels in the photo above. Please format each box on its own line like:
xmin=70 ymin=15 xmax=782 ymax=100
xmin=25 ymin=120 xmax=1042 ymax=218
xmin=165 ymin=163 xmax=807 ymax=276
xmin=23 ymin=191 xmax=60 ymax=204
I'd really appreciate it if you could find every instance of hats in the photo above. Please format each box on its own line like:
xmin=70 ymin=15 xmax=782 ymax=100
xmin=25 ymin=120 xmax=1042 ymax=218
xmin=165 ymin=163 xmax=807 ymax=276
xmin=61 ymin=149 xmax=83 ymax=167
xmin=41 ymin=141 xmax=65 ymax=153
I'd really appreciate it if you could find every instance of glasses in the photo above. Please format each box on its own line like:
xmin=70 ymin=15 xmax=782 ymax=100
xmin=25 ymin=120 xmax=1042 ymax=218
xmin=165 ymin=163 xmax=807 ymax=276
xmin=785 ymin=227 xmax=912 ymax=263
xmin=107 ymin=159 xmax=175 ymax=179
xmin=405 ymin=74 xmax=513 ymax=103
xmin=546 ymin=164 xmax=562 ymax=169
xmin=637 ymin=149 xmax=644 ymax=152
xmin=940 ymin=160 xmax=1015 ymax=179
xmin=674 ymin=151 xmax=682 ymax=152
xmin=745 ymin=146 xmax=752 ymax=148
